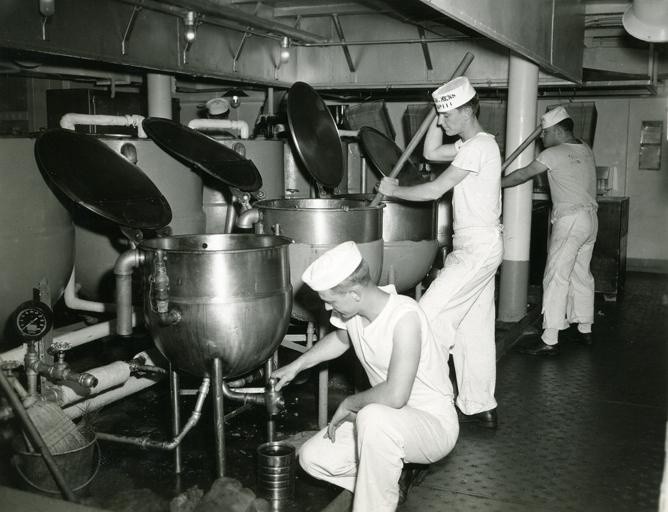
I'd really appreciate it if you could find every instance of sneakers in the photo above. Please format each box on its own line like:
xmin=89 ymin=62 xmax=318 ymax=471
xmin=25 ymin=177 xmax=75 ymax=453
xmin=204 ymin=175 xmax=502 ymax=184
xmin=399 ymin=467 xmax=415 ymax=504
xmin=561 ymin=328 xmax=593 ymax=345
xmin=520 ymin=339 xmax=560 ymax=356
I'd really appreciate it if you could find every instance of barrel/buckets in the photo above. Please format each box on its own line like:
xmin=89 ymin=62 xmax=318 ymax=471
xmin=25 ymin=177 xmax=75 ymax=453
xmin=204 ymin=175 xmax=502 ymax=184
xmin=256 ymin=441 xmax=296 ymax=505
xmin=12 ymin=429 xmax=101 ymax=500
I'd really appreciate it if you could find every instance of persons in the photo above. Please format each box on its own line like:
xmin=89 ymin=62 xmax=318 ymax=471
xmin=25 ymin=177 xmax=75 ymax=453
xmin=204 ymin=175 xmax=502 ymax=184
xmin=502 ymin=104 xmax=600 ymax=360
xmin=377 ymin=76 xmax=506 ymax=428
xmin=272 ymin=240 xmax=461 ymax=512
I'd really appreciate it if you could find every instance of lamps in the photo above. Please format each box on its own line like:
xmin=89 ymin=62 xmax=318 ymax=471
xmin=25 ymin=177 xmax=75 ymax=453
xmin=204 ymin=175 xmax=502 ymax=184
xmin=181 ymin=11 xmax=199 ymax=43
xmin=220 ymin=89 xmax=249 ymax=108
xmin=279 ymin=35 xmax=292 ymax=62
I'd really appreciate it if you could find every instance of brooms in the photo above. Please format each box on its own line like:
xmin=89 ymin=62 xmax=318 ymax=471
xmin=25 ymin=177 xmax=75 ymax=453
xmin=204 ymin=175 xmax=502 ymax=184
xmin=0 ymin=355 xmax=90 ymax=454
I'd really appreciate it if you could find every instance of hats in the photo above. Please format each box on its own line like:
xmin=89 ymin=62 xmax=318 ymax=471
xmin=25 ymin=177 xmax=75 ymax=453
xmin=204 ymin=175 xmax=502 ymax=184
xmin=301 ymin=239 xmax=363 ymax=293
xmin=430 ymin=76 xmax=476 ymax=114
xmin=540 ymin=105 xmax=569 ymax=129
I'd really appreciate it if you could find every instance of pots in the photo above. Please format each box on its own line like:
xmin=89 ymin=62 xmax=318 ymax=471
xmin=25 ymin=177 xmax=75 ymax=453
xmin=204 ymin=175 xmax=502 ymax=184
xmin=140 ymin=115 xmax=386 ymax=330
xmin=278 ymin=80 xmax=442 ymax=292
xmin=356 ymin=123 xmax=452 ymax=262
xmin=35 ymin=127 xmax=295 ymax=382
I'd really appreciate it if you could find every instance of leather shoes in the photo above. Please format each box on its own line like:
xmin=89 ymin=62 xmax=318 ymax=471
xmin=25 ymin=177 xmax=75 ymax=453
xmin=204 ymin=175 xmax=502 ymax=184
xmin=454 ymin=406 xmax=498 ymax=430
